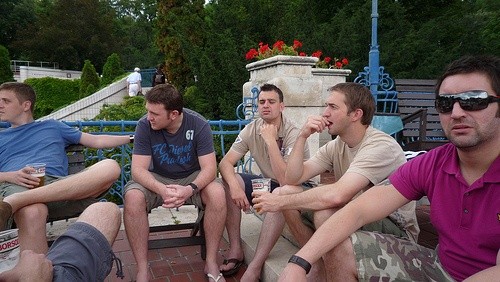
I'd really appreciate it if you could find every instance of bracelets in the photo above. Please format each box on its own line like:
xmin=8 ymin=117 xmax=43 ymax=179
xmin=288 ymin=255 xmax=312 ymax=274
xmin=129 ymin=134 xmax=134 ymax=144
xmin=139 ymin=92 xmax=142 ymax=94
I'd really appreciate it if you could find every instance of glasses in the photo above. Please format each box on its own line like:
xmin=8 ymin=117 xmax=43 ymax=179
xmin=434 ymin=90 xmax=500 ymax=114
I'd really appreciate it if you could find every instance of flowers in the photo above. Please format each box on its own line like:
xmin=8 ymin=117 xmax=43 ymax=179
xmin=246 ymin=39 xmax=348 ymax=71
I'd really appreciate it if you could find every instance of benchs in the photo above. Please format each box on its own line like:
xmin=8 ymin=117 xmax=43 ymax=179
xmin=396 ymin=79 xmax=451 ymax=153
xmin=37 ymin=144 xmax=95 ymax=221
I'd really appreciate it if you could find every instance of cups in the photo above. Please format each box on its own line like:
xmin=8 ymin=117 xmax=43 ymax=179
xmin=26 ymin=163 xmax=46 ymax=189
xmin=0 ymin=229 xmax=20 ymax=274
xmin=251 ymin=178 xmax=271 ymax=211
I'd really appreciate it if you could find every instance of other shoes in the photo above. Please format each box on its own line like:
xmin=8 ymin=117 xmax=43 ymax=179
xmin=0 ymin=196 xmax=12 ymax=232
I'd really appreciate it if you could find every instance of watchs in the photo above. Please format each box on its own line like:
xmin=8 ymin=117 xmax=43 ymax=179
xmin=187 ymin=183 xmax=199 ymax=195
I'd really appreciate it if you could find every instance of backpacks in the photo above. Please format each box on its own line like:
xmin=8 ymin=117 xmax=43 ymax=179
xmin=154 ymin=71 xmax=163 ymax=82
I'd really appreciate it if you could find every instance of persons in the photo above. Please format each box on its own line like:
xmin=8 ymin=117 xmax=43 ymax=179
xmin=276 ymin=54 xmax=500 ymax=282
xmin=217 ymin=84 xmax=320 ymax=282
xmin=251 ymin=81 xmax=420 ymax=282
xmin=0 ymin=83 xmax=135 ymax=255
xmin=127 ymin=67 xmax=142 ymax=98
xmin=152 ymin=64 xmax=165 ymax=87
xmin=122 ymin=83 xmax=225 ymax=282
xmin=0 ymin=202 xmax=122 ymax=282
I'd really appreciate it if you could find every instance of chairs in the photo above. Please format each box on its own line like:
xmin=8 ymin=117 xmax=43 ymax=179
xmin=147 ymin=208 xmax=207 ymax=262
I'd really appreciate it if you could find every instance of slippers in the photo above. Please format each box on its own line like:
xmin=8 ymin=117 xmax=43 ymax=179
xmin=219 ymin=258 xmax=244 ymax=276
xmin=205 ymin=272 xmax=227 ymax=282
